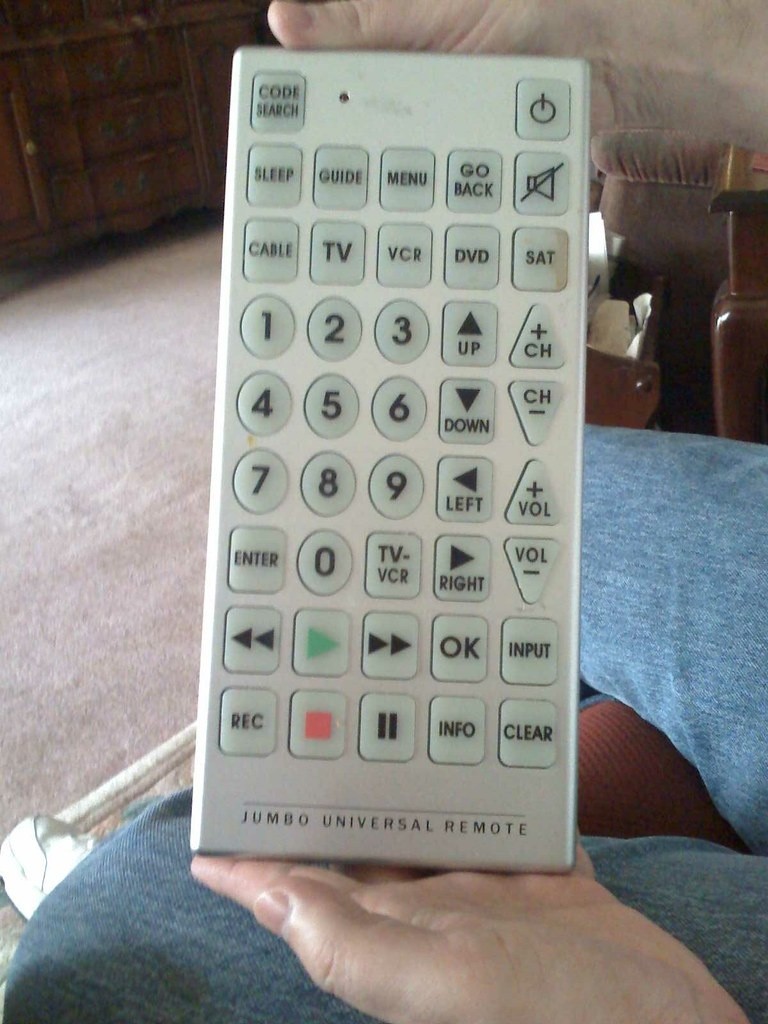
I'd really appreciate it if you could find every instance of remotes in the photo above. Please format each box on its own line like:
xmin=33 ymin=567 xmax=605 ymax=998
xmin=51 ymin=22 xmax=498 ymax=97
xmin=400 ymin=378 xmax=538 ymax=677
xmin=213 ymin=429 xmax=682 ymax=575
xmin=187 ymin=43 xmax=592 ymax=878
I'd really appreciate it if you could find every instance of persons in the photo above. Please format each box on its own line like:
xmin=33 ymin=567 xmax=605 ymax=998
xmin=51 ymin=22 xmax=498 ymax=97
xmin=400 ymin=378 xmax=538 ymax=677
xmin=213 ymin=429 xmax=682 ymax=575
xmin=0 ymin=0 xmax=768 ymax=1024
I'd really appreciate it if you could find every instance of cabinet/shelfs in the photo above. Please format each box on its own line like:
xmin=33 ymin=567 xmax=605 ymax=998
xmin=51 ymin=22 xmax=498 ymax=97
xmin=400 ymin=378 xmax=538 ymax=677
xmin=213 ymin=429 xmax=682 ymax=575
xmin=0 ymin=1 xmax=284 ymax=271
